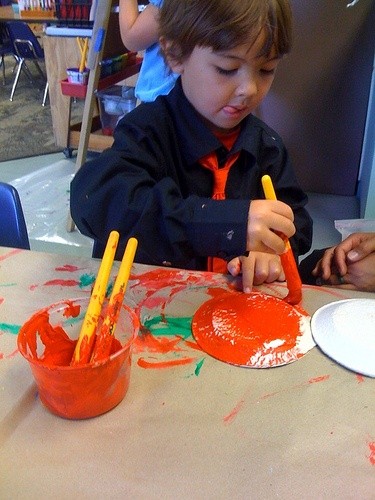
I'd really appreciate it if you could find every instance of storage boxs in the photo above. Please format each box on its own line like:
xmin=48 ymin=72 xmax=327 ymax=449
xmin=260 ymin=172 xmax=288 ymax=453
xmin=55 ymin=0 xmax=94 ymax=29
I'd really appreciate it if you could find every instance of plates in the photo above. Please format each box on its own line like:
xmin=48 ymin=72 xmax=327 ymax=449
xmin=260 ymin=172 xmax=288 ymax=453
xmin=192 ymin=293 xmax=316 ymax=369
xmin=309 ymin=299 xmax=375 ymax=378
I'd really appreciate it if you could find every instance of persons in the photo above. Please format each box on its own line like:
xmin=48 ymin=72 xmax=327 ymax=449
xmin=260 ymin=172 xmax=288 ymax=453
xmin=299 ymin=231 xmax=375 ymax=293
xmin=69 ymin=0 xmax=315 ymax=294
xmin=118 ymin=0 xmax=181 ymax=103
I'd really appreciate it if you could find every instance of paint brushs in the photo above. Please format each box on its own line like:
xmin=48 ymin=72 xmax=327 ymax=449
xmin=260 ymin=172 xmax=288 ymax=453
xmin=261 ymin=173 xmax=304 ymax=304
xmin=79 ymin=37 xmax=88 ymax=73
xmin=71 ymin=230 xmax=120 ymax=367
xmin=77 ymin=36 xmax=88 ymax=68
xmin=91 ymin=235 xmax=138 ymax=365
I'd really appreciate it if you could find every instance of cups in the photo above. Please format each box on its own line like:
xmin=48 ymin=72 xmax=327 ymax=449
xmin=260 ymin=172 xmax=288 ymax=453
xmin=18 ymin=298 xmax=138 ymax=421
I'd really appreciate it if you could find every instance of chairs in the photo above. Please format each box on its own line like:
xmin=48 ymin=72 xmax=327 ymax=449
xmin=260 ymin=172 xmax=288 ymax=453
xmin=0 ymin=21 xmax=48 ymax=107
xmin=0 ymin=182 xmax=30 ymax=251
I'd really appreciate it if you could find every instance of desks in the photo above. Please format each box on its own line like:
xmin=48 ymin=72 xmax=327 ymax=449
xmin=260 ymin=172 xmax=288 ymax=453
xmin=0 ymin=4 xmax=61 ymax=50
xmin=0 ymin=246 xmax=375 ymax=500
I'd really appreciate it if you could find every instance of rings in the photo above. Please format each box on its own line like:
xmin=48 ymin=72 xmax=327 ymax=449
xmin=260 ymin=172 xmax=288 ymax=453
xmin=337 ymin=274 xmax=344 ymax=284
